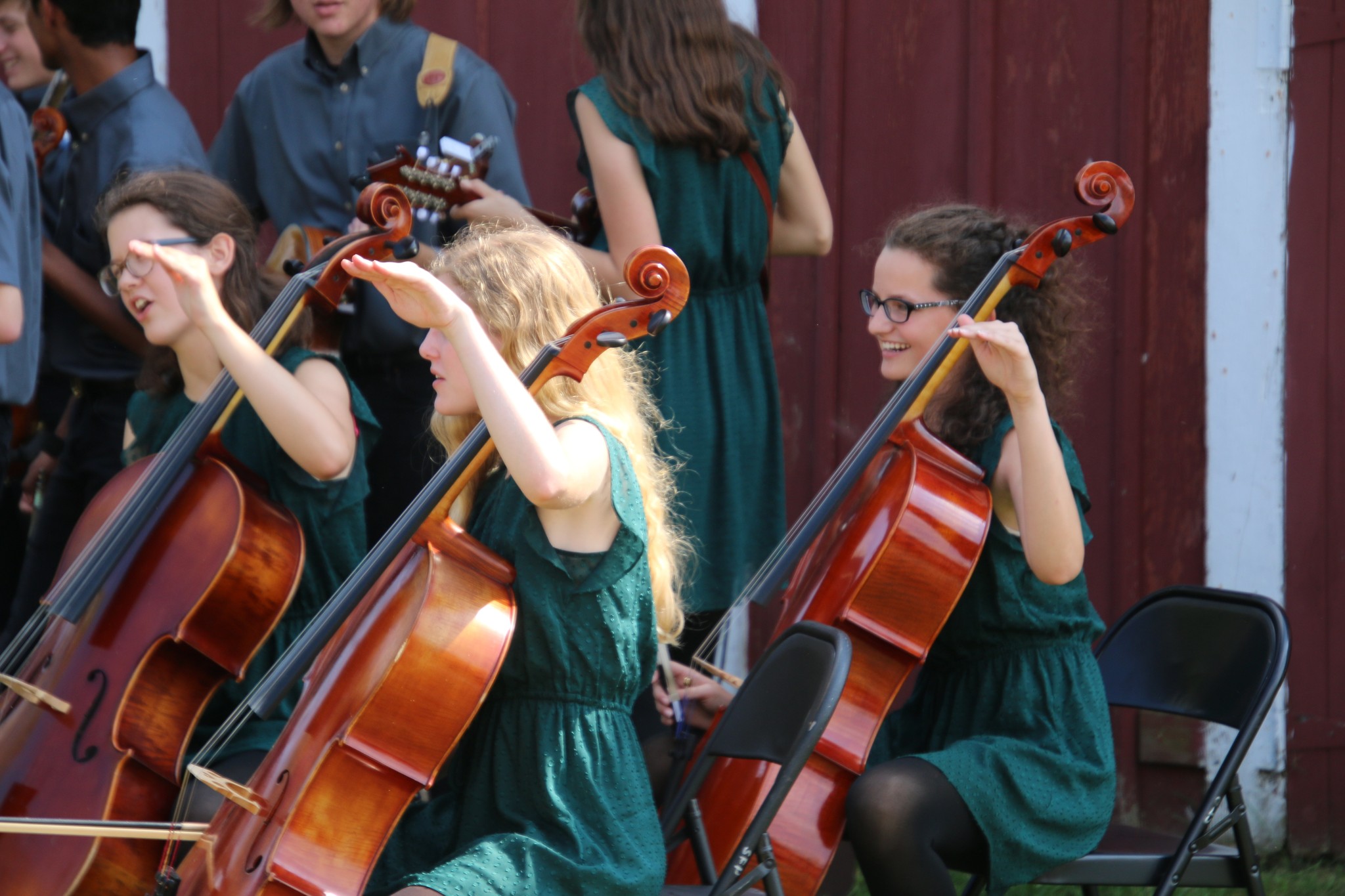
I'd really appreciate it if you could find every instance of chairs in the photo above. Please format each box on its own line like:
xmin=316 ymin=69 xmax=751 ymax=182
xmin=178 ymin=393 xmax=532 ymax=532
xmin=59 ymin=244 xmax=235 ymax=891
xmin=957 ymin=584 xmax=1291 ymax=896
xmin=662 ymin=620 xmax=852 ymax=896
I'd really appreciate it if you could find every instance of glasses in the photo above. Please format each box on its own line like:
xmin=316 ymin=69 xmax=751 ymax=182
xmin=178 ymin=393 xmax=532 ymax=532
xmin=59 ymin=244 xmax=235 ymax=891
xmin=858 ymin=286 xmax=969 ymax=323
xmin=97 ymin=236 xmax=196 ymax=297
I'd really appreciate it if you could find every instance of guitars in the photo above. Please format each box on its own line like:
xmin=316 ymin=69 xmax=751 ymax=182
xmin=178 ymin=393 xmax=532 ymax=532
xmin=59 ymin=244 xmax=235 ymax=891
xmin=363 ymin=132 xmax=600 ymax=248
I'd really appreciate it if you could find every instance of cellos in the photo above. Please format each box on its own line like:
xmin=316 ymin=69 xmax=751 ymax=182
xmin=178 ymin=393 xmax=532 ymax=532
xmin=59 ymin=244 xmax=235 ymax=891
xmin=0 ymin=176 xmax=413 ymax=896
xmin=660 ymin=158 xmax=1141 ymax=896
xmin=152 ymin=245 xmax=692 ymax=896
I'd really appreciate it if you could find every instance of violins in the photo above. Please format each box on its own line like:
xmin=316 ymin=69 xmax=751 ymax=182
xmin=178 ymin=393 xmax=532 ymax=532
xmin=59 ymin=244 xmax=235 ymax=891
xmin=32 ymin=106 xmax=67 ymax=176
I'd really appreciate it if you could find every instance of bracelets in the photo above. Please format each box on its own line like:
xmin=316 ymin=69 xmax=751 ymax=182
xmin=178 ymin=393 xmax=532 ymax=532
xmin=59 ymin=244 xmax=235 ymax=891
xmin=43 ymin=432 xmax=67 ymax=459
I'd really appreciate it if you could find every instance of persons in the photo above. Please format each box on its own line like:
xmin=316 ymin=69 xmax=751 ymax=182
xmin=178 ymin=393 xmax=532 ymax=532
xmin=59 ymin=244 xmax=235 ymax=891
xmin=0 ymin=0 xmax=532 ymax=692
xmin=652 ymin=200 xmax=1119 ymax=896
xmin=550 ymin=0 xmax=844 ymax=618
xmin=170 ymin=216 xmax=692 ymax=896
